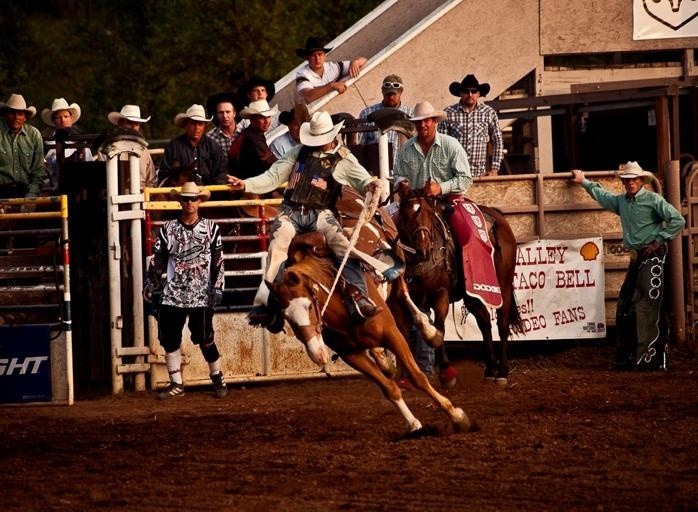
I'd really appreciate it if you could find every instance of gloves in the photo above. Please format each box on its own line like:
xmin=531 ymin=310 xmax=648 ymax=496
xmin=142 ymin=283 xmax=154 ymax=303
xmin=209 ymin=287 xmax=223 ymax=307
xmin=20 ymin=192 xmax=37 ymax=213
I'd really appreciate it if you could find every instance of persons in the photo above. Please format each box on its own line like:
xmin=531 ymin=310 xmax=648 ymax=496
xmin=0 ymin=94 xmax=43 ymax=213
xmin=204 ymin=91 xmax=244 ymax=176
xmin=227 ymin=100 xmax=278 ymax=179
xmin=391 ymin=102 xmax=473 ymax=196
xmin=225 ymin=111 xmax=384 ymax=320
xmin=295 ymin=37 xmax=368 ymax=105
xmin=141 ymin=181 xmax=228 ymax=399
xmin=237 ymin=75 xmax=284 ymax=134
xmin=438 ymin=73 xmax=504 ymax=178
xmin=41 ymin=97 xmax=94 ymax=191
xmin=571 ymin=161 xmax=686 ymax=373
xmin=359 ymin=75 xmax=416 ymax=178
xmin=270 ymin=106 xmax=314 ymax=161
xmin=107 ymin=103 xmax=158 ymax=196
xmin=158 ymin=104 xmax=225 ymax=201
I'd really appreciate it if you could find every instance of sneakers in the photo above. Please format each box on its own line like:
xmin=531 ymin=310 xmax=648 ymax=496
xmin=248 ymin=308 xmax=285 ymax=334
xmin=352 ymin=304 xmax=383 ymax=324
xmin=155 ymin=382 xmax=186 ymax=402
xmin=209 ymin=370 xmax=228 ymax=398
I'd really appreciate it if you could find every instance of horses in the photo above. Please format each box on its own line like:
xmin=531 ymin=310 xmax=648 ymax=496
xmin=193 ymin=169 xmax=283 ymax=306
xmin=150 ymin=154 xmax=203 ymax=239
xmin=264 ymin=88 xmax=481 ymax=438
xmin=384 ymin=175 xmax=526 ymax=393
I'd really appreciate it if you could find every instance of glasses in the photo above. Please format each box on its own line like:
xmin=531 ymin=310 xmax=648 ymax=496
xmin=462 ymin=88 xmax=478 ymax=94
xmin=383 ymin=81 xmax=401 ymax=88
xmin=181 ymin=196 xmax=199 ymax=203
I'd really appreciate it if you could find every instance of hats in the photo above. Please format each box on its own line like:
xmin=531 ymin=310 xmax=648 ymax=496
xmin=408 ymin=101 xmax=448 ymax=121
xmin=170 ymin=181 xmax=211 ymax=201
xmin=295 ymin=36 xmax=333 ymax=59
xmin=0 ymin=93 xmax=38 ymax=119
xmin=174 ymin=103 xmax=214 ymax=129
xmin=237 ymin=75 xmax=275 ymax=103
xmin=299 ymin=110 xmax=346 ymax=147
xmin=206 ymin=91 xmax=244 ymax=127
xmin=618 ymin=161 xmax=653 ymax=185
xmin=381 ymin=74 xmax=404 ymax=94
xmin=239 ymin=99 xmax=279 ymax=119
xmin=40 ymin=98 xmax=82 ymax=127
xmin=278 ymin=103 xmax=312 ymax=126
xmin=108 ymin=104 xmax=151 ymax=126
xmin=449 ymin=74 xmax=490 ymax=97
